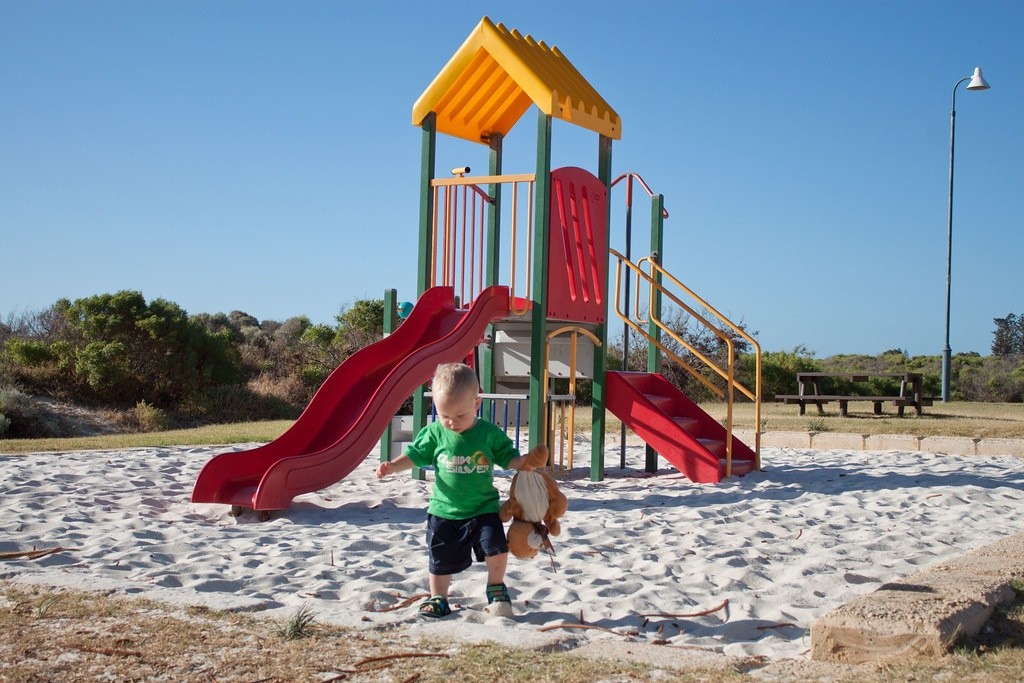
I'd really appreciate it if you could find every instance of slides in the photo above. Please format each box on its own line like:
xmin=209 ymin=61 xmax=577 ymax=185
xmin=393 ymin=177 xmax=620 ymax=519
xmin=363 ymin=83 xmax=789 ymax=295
xmin=188 ymin=285 xmax=511 ymax=513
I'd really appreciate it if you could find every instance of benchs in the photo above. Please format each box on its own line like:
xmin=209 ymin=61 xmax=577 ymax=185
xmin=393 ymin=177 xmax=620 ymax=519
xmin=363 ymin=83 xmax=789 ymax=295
xmin=775 ymin=394 xmax=907 ymax=407
xmin=909 ymin=396 xmax=944 ymax=407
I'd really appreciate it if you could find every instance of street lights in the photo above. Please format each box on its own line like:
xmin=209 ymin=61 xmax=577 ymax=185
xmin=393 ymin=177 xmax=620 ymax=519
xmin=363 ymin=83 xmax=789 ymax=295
xmin=940 ymin=67 xmax=991 ymax=403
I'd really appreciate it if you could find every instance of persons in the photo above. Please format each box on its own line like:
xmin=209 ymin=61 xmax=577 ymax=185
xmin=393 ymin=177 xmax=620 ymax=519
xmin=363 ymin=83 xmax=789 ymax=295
xmin=377 ymin=363 xmax=531 ymax=618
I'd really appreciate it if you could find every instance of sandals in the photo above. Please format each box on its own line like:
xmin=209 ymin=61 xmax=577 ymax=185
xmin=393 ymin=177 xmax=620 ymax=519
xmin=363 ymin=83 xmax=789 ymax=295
xmin=419 ymin=595 xmax=451 ymax=617
xmin=486 ymin=584 xmax=511 ymax=605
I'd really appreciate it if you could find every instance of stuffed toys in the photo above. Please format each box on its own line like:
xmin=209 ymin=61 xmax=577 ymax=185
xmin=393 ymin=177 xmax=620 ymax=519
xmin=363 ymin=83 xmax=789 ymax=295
xmin=498 ymin=445 xmax=568 ymax=559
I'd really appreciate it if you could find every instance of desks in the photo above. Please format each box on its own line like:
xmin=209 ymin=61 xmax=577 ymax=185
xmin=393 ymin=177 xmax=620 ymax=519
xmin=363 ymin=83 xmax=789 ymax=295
xmin=797 ymin=372 xmax=924 ymax=418
